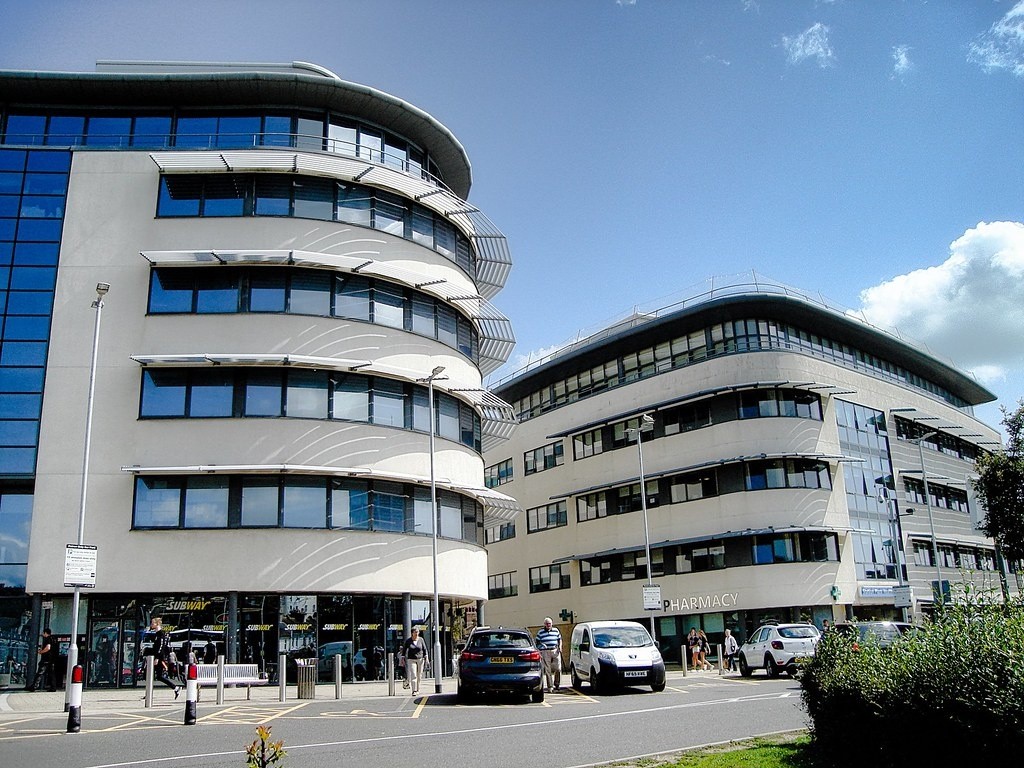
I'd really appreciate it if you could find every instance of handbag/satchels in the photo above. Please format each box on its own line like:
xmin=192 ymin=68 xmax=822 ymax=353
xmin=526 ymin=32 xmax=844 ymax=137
xmin=730 ymin=638 xmax=740 ymax=652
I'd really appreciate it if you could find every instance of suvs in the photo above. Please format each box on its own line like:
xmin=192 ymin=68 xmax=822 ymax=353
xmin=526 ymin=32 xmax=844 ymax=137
xmin=814 ymin=621 xmax=931 ymax=657
xmin=738 ymin=618 xmax=823 ymax=679
xmin=354 ymin=647 xmax=384 ymax=680
xmin=453 ymin=624 xmax=548 ymax=704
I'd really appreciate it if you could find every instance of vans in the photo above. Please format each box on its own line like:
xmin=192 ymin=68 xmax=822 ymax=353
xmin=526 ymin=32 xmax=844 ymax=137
xmin=569 ymin=620 xmax=666 ymax=695
xmin=318 ymin=640 xmax=354 ymax=673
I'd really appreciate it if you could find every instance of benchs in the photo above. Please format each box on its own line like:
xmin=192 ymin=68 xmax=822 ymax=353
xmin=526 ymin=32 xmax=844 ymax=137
xmin=185 ymin=664 xmax=269 ymax=700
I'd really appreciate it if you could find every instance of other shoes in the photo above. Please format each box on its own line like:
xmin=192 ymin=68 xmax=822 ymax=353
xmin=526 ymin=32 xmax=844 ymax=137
xmin=703 ymin=665 xmax=707 ymax=671
xmin=412 ymin=690 xmax=420 ymax=696
xmin=548 ymin=687 xmax=554 ymax=693
xmin=710 ymin=665 xmax=714 ymax=672
xmin=47 ymin=687 xmax=57 ymax=692
xmin=725 ymin=668 xmax=730 ymax=673
xmin=731 ymin=670 xmax=738 ymax=673
xmin=697 ymin=670 xmax=704 ymax=673
xmin=554 ymin=687 xmax=560 ymax=693
xmin=174 ymin=686 xmax=182 ymax=700
xmin=24 ymin=686 xmax=35 ymax=692
xmin=690 ymin=670 xmax=697 ymax=673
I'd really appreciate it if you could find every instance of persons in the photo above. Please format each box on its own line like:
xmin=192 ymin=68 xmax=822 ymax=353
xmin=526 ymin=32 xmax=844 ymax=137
xmin=140 ymin=617 xmax=182 ymax=700
xmin=32 ymin=628 xmax=58 ymax=692
xmin=724 ymin=628 xmax=739 ymax=671
xmin=823 ymin=619 xmax=831 ymax=631
xmin=309 ymin=643 xmax=316 ymax=659
xmin=88 ymin=634 xmax=226 ymax=688
xmin=536 ymin=616 xmax=562 ymax=693
xmin=397 ymin=626 xmax=430 ymax=697
xmin=687 ymin=627 xmax=714 ymax=672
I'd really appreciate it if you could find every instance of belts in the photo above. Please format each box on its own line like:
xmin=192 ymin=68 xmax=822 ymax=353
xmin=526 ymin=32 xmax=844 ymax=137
xmin=542 ymin=648 xmax=555 ymax=650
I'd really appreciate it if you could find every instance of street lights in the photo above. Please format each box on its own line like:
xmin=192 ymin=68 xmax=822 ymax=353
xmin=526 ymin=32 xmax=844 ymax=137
xmin=415 ymin=364 xmax=450 ymax=694
xmin=62 ymin=282 xmax=112 ymax=714
xmin=622 ymin=422 xmax=656 ymax=642
xmin=916 ymin=431 xmax=946 ymax=611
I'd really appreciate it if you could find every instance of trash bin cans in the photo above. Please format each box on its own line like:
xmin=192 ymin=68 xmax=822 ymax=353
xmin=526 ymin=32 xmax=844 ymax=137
xmin=294 ymin=658 xmax=319 ymax=699
